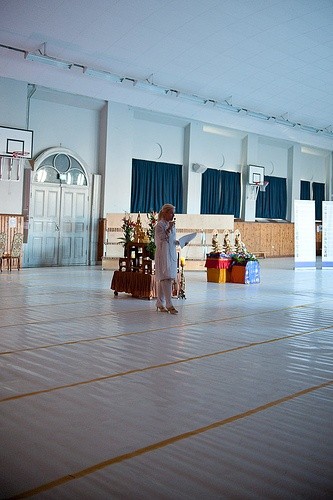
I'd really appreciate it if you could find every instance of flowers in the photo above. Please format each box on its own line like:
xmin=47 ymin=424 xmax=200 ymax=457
xmin=117 ymin=211 xmax=159 ymax=260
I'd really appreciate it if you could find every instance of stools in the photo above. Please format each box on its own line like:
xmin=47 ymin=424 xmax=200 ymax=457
xmin=119 ymin=243 xmax=155 ymax=275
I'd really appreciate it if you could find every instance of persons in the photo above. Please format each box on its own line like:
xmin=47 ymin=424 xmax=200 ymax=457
xmin=235 ymin=234 xmax=240 ymax=245
xmin=155 ymin=204 xmax=188 ymax=314
xmin=224 ymin=233 xmax=230 ymax=245
xmin=212 ymin=234 xmax=220 ymax=245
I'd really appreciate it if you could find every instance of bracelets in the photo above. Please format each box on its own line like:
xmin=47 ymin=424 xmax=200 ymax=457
xmin=167 ymin=229 xmax=171 ymax=233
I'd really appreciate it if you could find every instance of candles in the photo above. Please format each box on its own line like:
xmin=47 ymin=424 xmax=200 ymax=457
xmin=180 ymin=257 xmax=185 ymax=265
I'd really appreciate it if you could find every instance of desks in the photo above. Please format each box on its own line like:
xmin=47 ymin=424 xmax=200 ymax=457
xmin=111 ymin=271 xmax=181 ymax=300
xmin=205 ymin=253 xmax=257 ymax=283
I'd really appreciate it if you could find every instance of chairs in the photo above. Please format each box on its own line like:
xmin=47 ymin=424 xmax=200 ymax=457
xmin=0 ymin=232 xmax=24 ymax=272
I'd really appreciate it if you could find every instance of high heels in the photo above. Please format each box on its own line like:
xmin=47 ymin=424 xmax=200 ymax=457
xmin=165 ymin=306 xmax=178 ymax=314
xmin=156 ymin=304 xmax=167 ymax=312
